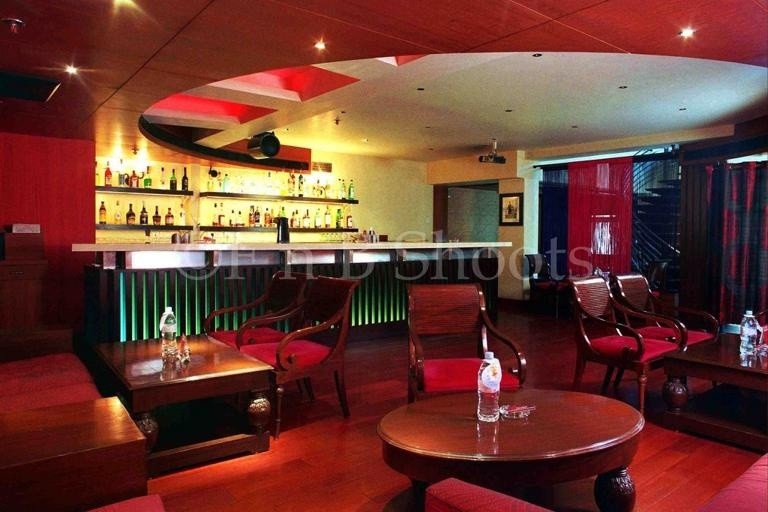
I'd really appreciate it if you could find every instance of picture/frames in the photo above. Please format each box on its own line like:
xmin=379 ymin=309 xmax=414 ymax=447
xmin=496 ymin=190 xmax=523 ymax=226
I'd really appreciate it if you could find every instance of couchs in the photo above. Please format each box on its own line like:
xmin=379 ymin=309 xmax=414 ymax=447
xmin=0 ymin=322 xmax=166 ymax=512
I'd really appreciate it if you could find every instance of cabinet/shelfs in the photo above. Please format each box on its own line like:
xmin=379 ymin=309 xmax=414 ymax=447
xmin=94 ymin=156 xmax=358 ymax=243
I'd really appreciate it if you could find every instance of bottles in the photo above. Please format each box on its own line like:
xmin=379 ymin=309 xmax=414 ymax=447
xmin=98 ymin=201 xmax=107 ymax=224
xmin=318 ymin=209 xmax=321 ymax=228
xmin=282 ymin=206 xmax=286 ymax=217
xmin=314 ymin=212 xmax=319 ymax=228
xmin=178 ymin=203 xmax=186 ymax=226
xmin=336 ymin=209 xmax=343 ymax=228
xmin=182 ymin=167 xmax=188 ymax=190
xmin=209 ymin=168 xmax=305 ymax=197
xmin=293 ymin=210 xmax=300 ymax=228
xmin=219 ymin=202 xmax=225 ymax=227
xmin=140 ymin=201 xmax=149 ymax=225
xmin=105 ymin=161 xmax=112 ymax=189
xmin=255 ymin=206 xmax=261 ymax=228
xmin=114 ymin=200 xmax=123 ymax=225
xmin=128 ymin=170 xmax=140 ymax=188
xmin=164 ymin=208 xmax=174 ymax=226
xmin=95 ymin=161 xmax=101 ymax=186
xmin=306 ymin=209 xmax=310 ymax=229
xmin=118 ymin=159 xmax=125 ymax=187
xmin=212 ymin=203 xmax=219 ymax=226
xmin=738 ymin=310 xmax=758 ymax=369
xmin=312 ymin=177 xmax=355 ymax=200
xmin=249 ymin=205 xmax=255 ymax=227
xmin=152 ymin=206 xmax=161 ymax=226
xmin=277 ymin=206 xmax=282 ymax=218
xmin=264 ymin=208 xmax=271 ymax=228
xmin=161 ymin=306 xmax=179 ymax=365
xmin=302 ymin=214 xmax=306 ymax=229
xmin=236 ymin=210 xmax=245 ymax=227
xmin=126 ymin=203 xmax=136 ymax=225
xmin=476 ymin=350 xmax=500 ymax=423
xmin=229 ymin=209 xmax=236 ymax=227
xmin=143 ymin=165 xmax=152 ymax=189
xmin=169 ymin=168 xmax=177 ymax=190
xmin=159 ymin=166 xmax=167 ymax=189
xmin=270 ymin=209 xmax=274 ymax=228
xmin=325 ymin=205 xmax=331 ymax=228
xmin=290 ymin=211 xmax=295 ymax=227
xmin=346 ymin=208 xmax=353 ymax=229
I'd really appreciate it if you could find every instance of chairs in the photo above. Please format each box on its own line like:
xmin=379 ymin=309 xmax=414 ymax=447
xmin=570 ymin=274 xmax=684 ymax=422
xmin=612 ymin=271 xmax=722 ymax=399
xmin=234 ymin=274 xmax=351 ymax=421
xmin=403 ymin=281 xmax=525 ymax=403
xmin=203 ymin=270 xmax=316 ymax=407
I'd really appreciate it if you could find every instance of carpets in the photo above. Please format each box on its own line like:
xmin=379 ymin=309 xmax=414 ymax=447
xmin=145 ymin=308 xmax=768 ymax=510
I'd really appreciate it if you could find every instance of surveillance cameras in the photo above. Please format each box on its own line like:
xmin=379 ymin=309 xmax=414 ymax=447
xmin=480 ymin=155 xmax=508 ymax=166
